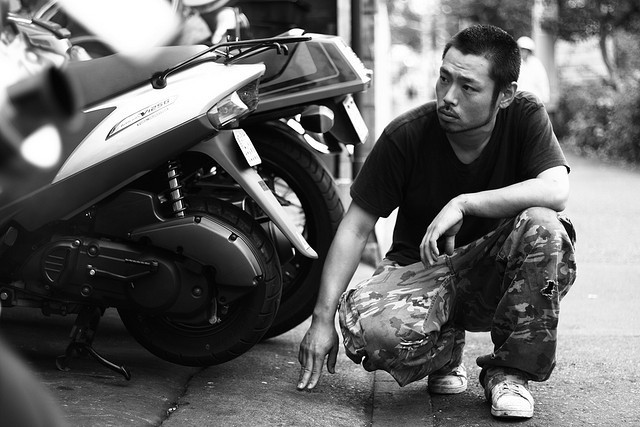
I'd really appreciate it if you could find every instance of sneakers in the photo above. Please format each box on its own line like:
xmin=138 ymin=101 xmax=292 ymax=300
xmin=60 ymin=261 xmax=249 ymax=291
xmin=482 ymin=369 xmax=535 ymax=421
xmin=428 ymin=364 xmax=469 ymax=396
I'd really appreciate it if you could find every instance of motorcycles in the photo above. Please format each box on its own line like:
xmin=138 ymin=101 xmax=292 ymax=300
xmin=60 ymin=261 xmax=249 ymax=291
xmin=0 ymin=44 xmax=312 ymax=380
xmin=188 ymin=28 xmax=373 ymax=338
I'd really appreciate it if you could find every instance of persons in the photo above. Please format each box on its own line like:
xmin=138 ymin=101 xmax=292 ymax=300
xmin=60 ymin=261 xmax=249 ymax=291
xmin=516 ymin=36 xmax=550 ymax=104
xmin=299 ymin=24 xmax=577 ymax=418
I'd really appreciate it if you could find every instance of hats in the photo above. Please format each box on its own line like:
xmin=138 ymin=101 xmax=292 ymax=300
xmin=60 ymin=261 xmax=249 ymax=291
xmin=517 ymin=36 xmax=535 ymax=54
